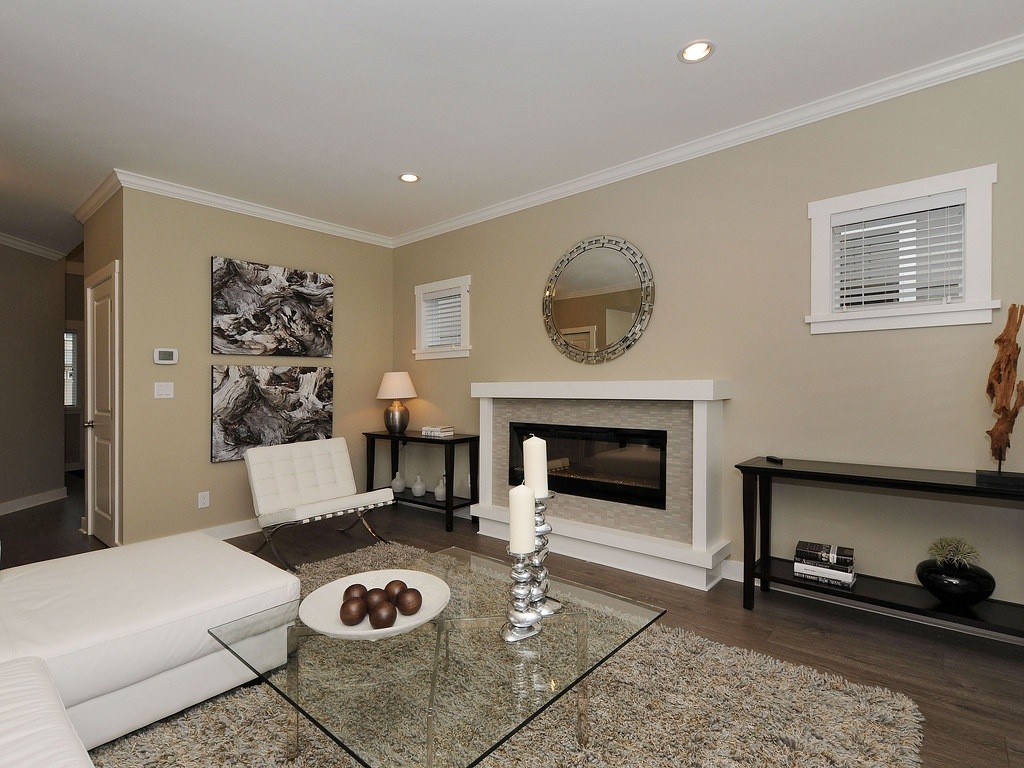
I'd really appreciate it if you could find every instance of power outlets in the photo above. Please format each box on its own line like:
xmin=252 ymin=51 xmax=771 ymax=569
xmin=198 ymin=491 xmax=209 ymax=508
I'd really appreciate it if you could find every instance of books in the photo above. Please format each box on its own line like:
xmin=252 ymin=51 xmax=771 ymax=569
xmin=422 ymin=425 xmax=454 ymax=436
xmin=793 ymin=540 xmax=857 ymax=588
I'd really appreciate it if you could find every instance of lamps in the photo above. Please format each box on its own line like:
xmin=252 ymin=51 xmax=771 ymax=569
xmin=376 ymin=371 xmax=418 ymax=433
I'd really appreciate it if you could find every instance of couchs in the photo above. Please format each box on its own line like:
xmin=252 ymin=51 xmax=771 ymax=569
xmin=0 ymin=531 xmax=302 ymax=768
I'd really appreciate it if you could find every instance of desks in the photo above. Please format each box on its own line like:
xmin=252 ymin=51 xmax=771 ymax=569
xmin=735 ymin=456 xmax=1024 ymax=647
xmin=362 ymin=430 xmax=479 ymax=531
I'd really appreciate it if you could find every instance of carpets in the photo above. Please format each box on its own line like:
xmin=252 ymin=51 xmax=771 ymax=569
xmin=86 ymin=541 xmax=926 ymax=767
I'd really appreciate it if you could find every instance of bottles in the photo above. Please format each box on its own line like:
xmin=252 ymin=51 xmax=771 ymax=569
xmin=390 ymin=472 xmax=446 ymax=501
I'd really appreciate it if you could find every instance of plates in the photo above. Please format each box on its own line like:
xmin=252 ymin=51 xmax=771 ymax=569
xmin=299 ymin=568 xmax=451 ymax=641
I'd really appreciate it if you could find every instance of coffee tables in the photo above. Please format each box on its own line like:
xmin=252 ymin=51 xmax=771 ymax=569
xmin=208 ymin=545 xmax=668 ymax=767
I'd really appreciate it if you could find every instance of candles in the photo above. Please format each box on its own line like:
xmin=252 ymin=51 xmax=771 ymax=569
xmin=508 ymin=480 xmax=535 ymax=554
xmin=523 ymin=433 xmax=549 ymax=498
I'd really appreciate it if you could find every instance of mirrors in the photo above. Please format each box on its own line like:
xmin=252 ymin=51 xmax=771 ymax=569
xmin=542 ymin=235 xmax=654 ymax=366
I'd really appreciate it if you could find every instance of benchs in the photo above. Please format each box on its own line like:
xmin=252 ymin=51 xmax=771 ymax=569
xmin=242 ymin=437 xmax=396 ymax=565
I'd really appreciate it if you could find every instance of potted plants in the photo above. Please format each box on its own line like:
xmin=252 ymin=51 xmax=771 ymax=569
xmin=916 ymin=536 xmax=995 ymax=608
